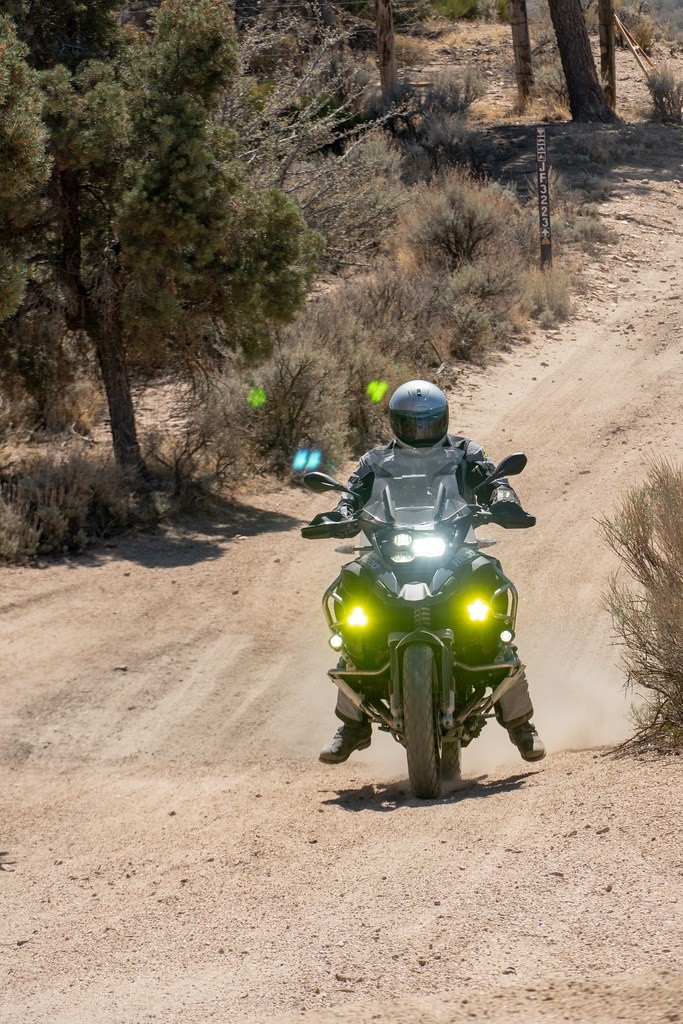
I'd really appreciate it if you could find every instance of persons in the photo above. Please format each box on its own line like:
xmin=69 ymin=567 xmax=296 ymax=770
xmin=316 ymin=379 xmax=550 ymax=763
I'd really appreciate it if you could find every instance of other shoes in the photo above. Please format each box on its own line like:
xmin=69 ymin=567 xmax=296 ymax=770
xmin=509 ymin=721 xmax=547 ymax=762
xmin=319 ymin=725 xmax=372 ymax=764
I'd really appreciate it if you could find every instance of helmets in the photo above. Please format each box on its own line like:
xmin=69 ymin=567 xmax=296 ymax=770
xmin=388 ymin=380 xmax=449 ymax=457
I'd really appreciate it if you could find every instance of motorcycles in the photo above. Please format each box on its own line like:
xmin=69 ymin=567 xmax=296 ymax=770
xmin=303 ymin=454 xmax=537 ymax=798
